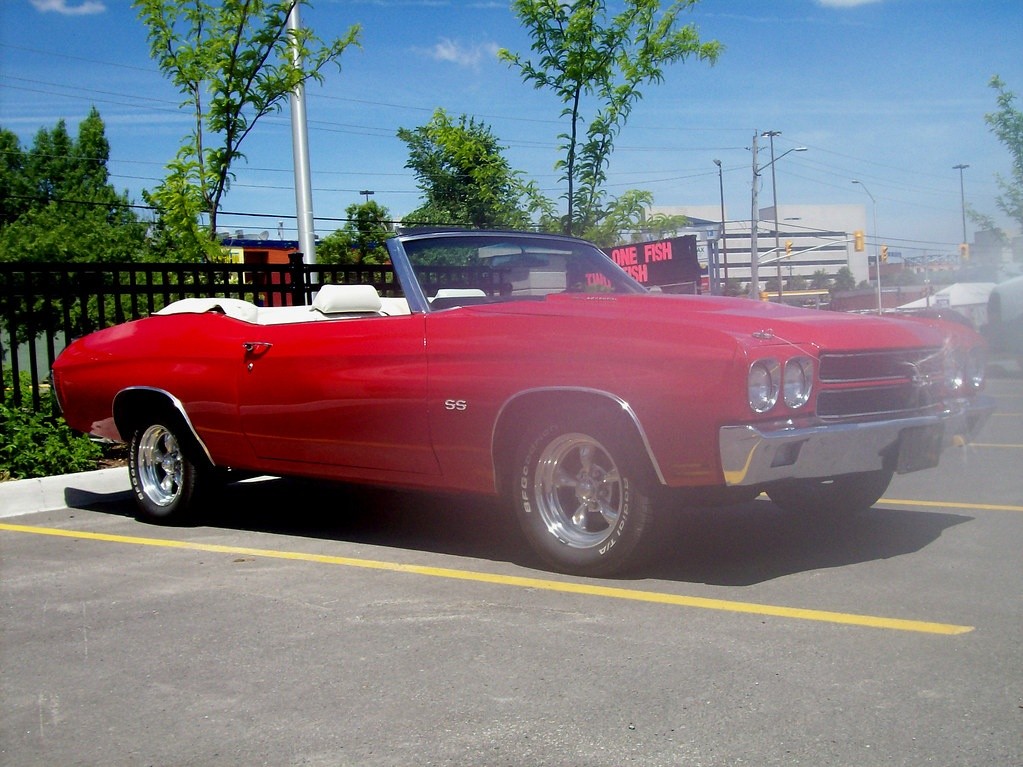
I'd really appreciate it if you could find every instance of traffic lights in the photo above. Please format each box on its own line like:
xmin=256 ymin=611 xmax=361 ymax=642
xmin=961 ymin=244 xmax=968 ymax=260
xmin=882 ymin=246 xmax=888 ymax=261
xmin=785 ymin=241 xmax=793 ymax=255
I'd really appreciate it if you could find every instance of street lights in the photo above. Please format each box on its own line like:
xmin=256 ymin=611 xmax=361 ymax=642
xmin=713 ymin=159 xmax=729 ymax=297
xmin=752 ymin=147 xmax=807 ymax=299
xmin=359 ymin=190 xmax=374 ymax=202
xmin=852 ymin=180 xmax=882 ymax=316
xmin=761 ymin=130 xmax=783 ymax=304
xmin=952 ymin=164 xmax=970 ymax=243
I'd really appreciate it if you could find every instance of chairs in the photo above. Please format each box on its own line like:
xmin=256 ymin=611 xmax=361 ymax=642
xmin=435 ymin=289 xmax=486 ymax=298
xmin=310 ymin=283 xmax=383 ymax=321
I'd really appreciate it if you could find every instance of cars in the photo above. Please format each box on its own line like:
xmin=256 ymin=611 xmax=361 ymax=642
xmin=911 ymin=309 xmax=966 ymax=323
xmin=51 ymin=227 xmax=997 ymax=578
